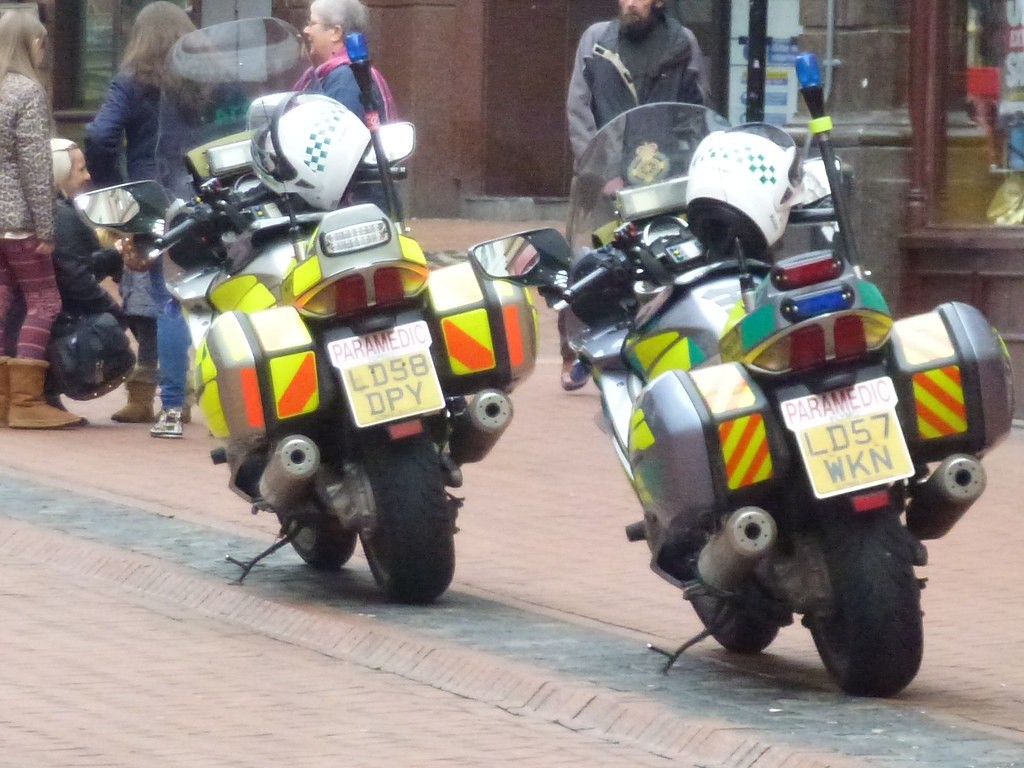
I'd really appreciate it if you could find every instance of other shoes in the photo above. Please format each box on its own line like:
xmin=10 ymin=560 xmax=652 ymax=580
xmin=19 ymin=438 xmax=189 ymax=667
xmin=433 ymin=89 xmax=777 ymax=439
xmin=560 ymin=363 xmax=590 ymax=390
xmin=44 ymin=394 xmax=90 ymax=427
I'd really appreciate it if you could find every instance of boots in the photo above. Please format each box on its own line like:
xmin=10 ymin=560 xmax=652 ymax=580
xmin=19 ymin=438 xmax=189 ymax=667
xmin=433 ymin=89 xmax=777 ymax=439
xmin=155 ymin=370 xmax=196 ymax=423
xmin=111 ymin=365 xmax=157 ymax=423
xmin=7 ymin=358 xmax=81 ymax=428
xmin=0 ymin=356 xmax=12 ymax=429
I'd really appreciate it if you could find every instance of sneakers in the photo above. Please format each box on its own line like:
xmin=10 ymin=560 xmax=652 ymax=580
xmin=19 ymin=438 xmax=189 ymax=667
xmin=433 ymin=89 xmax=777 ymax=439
xmin=150 ymin=408 xmax=183 ymax=439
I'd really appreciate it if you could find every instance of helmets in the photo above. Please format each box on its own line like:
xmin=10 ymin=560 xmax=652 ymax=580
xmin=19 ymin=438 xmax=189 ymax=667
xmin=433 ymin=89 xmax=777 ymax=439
xmin=685 ymin=123 xmax=807 ymax=259
xmin=246 ymin=93 xmax=373 ymax=211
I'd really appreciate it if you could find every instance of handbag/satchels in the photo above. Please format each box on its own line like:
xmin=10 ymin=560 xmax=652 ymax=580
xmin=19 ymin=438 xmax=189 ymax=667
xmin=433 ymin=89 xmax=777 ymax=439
xmin=47 ymin=311 xmax=137 ymax=401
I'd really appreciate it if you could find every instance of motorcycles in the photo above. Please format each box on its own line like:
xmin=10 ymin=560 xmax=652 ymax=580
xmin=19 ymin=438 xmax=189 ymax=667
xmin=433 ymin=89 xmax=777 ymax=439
xmin=71 ymin=16 xmax=543 ymax=608
xmin=466 ymin=100 xmax=1017 ymax=700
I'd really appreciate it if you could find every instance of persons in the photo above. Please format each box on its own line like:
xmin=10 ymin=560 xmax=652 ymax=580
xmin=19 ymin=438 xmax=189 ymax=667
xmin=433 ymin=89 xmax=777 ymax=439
xmin=46 ymin=140 xmax=137 ymax=414
xmin=0 ymin=11 xmax=81 ymax=428
xmin=84 ymin=1 xmax=401 ymax=457
xmin=557 ymin=1 xmax=714 ymax=390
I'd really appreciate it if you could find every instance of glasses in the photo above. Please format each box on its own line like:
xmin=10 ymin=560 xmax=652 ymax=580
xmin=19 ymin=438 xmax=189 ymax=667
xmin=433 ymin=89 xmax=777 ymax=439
xmin=305 ymin=20 xmax=334 ymax=29
